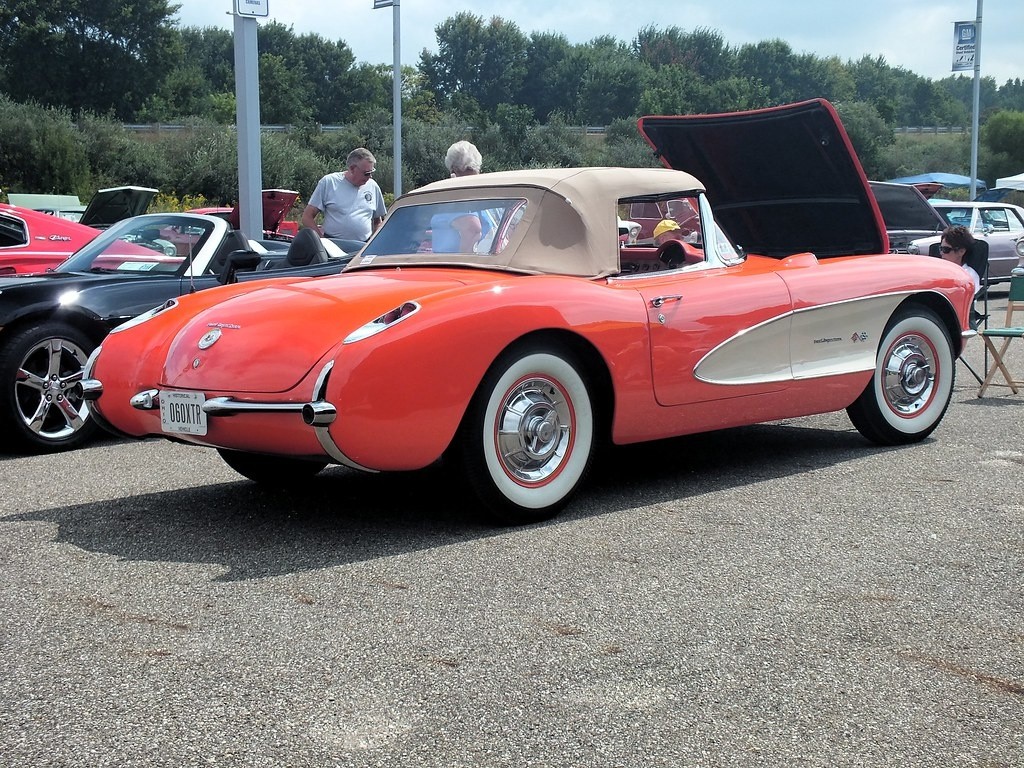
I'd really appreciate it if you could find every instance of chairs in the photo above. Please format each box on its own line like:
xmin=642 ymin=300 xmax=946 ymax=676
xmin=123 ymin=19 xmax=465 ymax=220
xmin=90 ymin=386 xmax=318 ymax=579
xmin=269 ymin=229 xmax=328 ymax=270
xmin=977 ymin=276 xmax=1024 ymax=399
xmin=206 ymin=231 xmax=257 ymax=276
xmin=928 ymin=239 xmax=989 ymax=385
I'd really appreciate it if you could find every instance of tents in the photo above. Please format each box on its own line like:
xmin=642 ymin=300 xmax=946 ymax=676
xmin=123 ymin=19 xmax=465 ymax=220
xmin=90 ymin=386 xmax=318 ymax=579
xmin=996 ymin=173 xmax=1024 ymax=190
xmin=885 ymin=172 xmax=986 ymax=188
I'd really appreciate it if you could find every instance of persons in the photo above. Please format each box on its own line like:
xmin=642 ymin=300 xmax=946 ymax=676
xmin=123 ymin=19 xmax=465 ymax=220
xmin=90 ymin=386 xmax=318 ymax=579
xmin=653 ymin=220 xmax=682 ymax=247
xmin=301 ymin=148 xmax=387 ymax=243
xmin=430 ymin=141 xmax=502 ymax=253
xmin=939 ymin=226 xmax=980 ymax=295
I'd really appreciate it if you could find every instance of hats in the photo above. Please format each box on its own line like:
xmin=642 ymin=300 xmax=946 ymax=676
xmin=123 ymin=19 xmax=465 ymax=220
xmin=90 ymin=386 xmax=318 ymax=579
xmin=653 ymin=220 xmax=681 ymax=239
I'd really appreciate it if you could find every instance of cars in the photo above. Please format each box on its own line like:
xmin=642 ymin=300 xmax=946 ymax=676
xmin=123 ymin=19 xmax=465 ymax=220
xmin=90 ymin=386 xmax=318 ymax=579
xmin=71 ymin=93 xmax=978 ymax=524
xmin=868 ymin=180 xmax=1024 ymax=285
xmin=0 ymin=209 xmax=368 ymax=454
xmin=4 ymin=182 xmax=298 ymax=243
xmin=1 ymin=201 xmax=199 ymax=277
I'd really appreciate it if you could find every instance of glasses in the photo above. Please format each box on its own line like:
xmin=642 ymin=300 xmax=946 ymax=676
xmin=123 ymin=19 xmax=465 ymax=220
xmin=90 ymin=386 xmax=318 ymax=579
xmin=357 ymin=166 xmax=376 ymax=176
xmin=939 ymin=245 xmax=954 ymax=254
